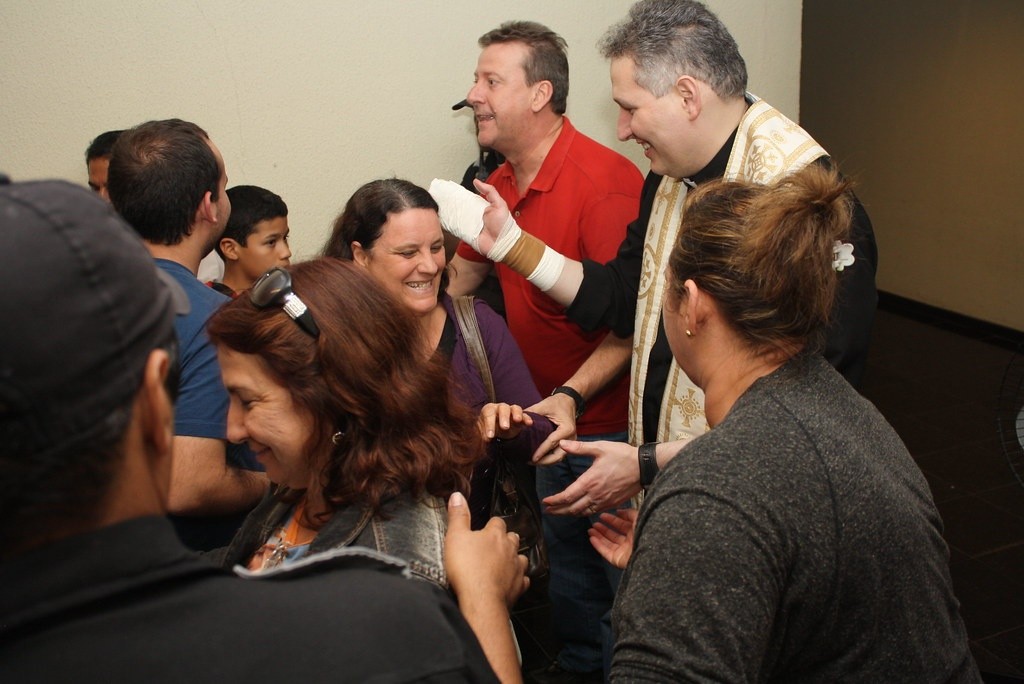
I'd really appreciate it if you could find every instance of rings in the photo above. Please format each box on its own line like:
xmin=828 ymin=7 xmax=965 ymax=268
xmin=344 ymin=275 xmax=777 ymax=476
xmin=589 ymin=506 xmax=597 ymax=514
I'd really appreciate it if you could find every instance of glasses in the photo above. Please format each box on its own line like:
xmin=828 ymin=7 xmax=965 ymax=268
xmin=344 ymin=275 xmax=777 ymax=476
xmin=249 ymin=265 xmax=319 ymax=339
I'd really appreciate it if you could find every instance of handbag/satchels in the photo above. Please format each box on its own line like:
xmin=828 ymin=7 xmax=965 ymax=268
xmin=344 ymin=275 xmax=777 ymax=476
xmin=453 ymin=295 xmax=551 ymax=594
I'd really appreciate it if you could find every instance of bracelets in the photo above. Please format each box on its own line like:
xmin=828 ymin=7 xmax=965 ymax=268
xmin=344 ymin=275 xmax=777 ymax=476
xmin=269 ymin=481 xmax=278 ymax=496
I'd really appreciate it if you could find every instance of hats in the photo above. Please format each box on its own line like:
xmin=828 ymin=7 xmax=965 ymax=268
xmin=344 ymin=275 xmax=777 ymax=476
xmin=0 ymin=179 xmax=191 ymax=462
xmin=452 ymin=99 xmax=473 ymax=111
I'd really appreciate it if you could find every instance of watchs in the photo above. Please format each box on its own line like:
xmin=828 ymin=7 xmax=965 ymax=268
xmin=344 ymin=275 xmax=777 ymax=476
xmin=638 ymin=442 xmax=661 ymax=489
xmin=551 ymin=386 xmax=587 ymax=421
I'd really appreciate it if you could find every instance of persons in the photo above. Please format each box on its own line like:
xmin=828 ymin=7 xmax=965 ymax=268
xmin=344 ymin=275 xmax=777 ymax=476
xmin=0 ymin=180 xmax=530 ymax=684
xmin=587 ymin=179 xmax=983 ymax=684
xmin=205 ymin=255 xmax=482 ymax=592
xmin=86 ymin=130 xmax=124 ymax=204
xmin=107 ymin=118 xmax=278 ymax=552
xmin=325 ymin=178 xmax=556 ymax=459
xmin=446 ymin=22 xmax=645 ymax=465
xmin=537 ymin=0 xmax=880 ymax=518
xmin=205 ymin=185 xmax=292 ymax=299
